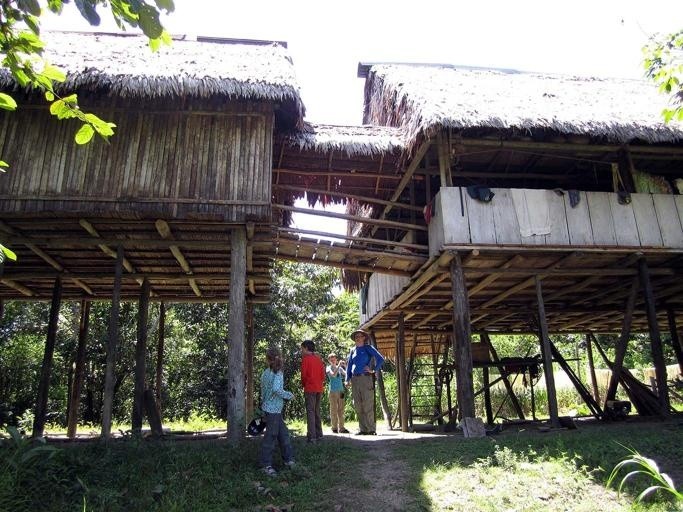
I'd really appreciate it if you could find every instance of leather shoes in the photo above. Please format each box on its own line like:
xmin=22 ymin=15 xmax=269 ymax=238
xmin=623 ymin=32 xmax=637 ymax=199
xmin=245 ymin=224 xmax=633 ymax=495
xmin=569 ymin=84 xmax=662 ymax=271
xmin=355 ymin=432 xmax=368 ymax=435
xmin=369 ymin=431 xmax=376 ymax=435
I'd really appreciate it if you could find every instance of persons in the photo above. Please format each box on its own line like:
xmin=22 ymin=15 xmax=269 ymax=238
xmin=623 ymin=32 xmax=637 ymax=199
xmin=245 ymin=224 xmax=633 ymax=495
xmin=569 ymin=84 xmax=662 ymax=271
xmin=259 ymin=348 xmax=298 ymax=477
xmin=327 ymin=353 xmax=348 ymax=433
xmin=300 ymin=341 xmax=326 ymax=443
xmin=345 ymin=328 xmax=386 ymax=436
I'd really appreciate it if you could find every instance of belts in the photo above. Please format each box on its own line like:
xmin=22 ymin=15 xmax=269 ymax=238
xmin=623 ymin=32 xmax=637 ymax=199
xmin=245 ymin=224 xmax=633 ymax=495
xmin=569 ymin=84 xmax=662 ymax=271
xmin=352 ymin=373 xmax=369 ymax=376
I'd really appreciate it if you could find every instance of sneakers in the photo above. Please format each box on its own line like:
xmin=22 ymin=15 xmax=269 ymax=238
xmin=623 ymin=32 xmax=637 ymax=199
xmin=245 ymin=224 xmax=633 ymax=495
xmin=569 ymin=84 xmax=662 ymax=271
xmin=333 ymin=430 xmax=338 ymax=433
xmin=339 ymin=430 xmax=348 ymax=433
xmin=261 ymin=466 xmax=278 ymax=478
xmin=283 ymin=461 xmax=295 ymax=467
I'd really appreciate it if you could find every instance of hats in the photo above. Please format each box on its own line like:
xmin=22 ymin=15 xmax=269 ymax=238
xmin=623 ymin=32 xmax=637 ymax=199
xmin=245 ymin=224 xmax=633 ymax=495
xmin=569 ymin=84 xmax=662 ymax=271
xmin=248 ymin=417 xmax=266 ymax=436
xmin=351 ymin=330 xmax=368 ymax=342
